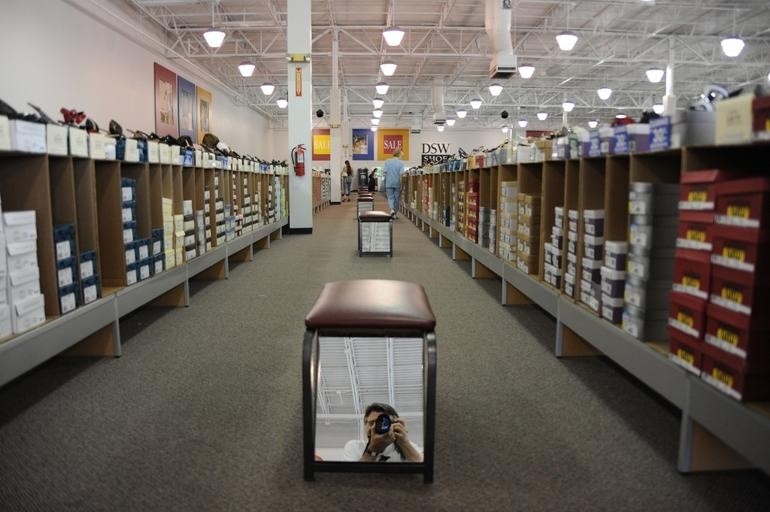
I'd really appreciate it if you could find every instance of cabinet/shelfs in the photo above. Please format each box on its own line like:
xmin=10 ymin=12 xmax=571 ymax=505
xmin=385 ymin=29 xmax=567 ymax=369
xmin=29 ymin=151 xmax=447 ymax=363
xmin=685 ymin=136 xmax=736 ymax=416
xmin=397 ymin=168 xmax=472 ymax=260
xmin=191 ymin=170 xmax=289 ymax=279
xmin=556 ymin=140 xmax=770 ymax=472
xmin=312 ymin=177 xmax=329 ymax=215
xmin=472 ymin=157 xmax=567 ymax=305
xmin=2 ymin=150 xmax=121 ymax=388
xmin=120 ymin=160 xmax=228 ymax=318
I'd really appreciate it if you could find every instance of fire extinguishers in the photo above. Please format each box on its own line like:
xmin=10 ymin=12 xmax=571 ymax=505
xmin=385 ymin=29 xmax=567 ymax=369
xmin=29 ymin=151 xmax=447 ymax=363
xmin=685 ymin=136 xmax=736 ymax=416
xmin=291 ymin=144 xmax=306 ymax=176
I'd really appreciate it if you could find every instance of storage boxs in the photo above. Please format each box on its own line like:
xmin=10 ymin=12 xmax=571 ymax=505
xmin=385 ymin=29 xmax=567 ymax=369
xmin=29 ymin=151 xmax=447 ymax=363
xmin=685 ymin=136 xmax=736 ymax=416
xmin=312 ymin=169 xmax=330 ymax=177
xmin=648 ymin=87 xmax=769 ymax=149
xmin=2 ymin=116 xmax=159 ymax=164
xmin=467 ymin=139 xmax=553 ymax=170
xmin=230 ymin=151 xmax=289 ymax=176
xmin=403 ymin=158 xmax=467 ymax=174
xmin=553 ymin=111 xmax=650 ymax=160
xmin=160 ymin=133 xmax=232 ymax=171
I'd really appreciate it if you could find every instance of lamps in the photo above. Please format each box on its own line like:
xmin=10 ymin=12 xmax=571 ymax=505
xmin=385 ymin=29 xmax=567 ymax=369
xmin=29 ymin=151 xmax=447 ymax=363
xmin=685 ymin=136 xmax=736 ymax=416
xmin=367 ymin=1 xmax=406 ymax=132
xmin=432 ymin=0 xmax=672 ymax=135
xmin=719 ymin=8 xmax=747 ymax=58
xmin=202 ymin=1 xmax=288 ymax=112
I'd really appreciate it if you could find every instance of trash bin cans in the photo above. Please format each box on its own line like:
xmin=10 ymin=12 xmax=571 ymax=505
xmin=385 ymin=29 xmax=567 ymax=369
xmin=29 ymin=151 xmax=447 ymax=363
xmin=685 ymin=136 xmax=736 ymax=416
xmin=359 ymin=168 xmax=369 ymax=187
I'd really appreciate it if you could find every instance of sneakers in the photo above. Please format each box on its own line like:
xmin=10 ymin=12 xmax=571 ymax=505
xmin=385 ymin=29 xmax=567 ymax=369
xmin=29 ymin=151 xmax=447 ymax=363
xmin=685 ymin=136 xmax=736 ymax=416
xmin=390 ymin=210 xmax=396 ymax=220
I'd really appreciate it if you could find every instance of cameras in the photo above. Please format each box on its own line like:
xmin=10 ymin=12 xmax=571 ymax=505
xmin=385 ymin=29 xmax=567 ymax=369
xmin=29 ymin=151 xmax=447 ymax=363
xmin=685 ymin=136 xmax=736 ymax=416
xmin=374 ymin=414 xmax=391 ymax=433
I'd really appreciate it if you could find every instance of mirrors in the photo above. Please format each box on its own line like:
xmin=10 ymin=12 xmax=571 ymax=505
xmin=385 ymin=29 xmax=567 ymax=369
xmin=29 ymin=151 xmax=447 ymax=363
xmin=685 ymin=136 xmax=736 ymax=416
xmin=359 ymin=219 xmax=392 ymax=255
xmin=300 ymin=324 xmax=429 ymax=469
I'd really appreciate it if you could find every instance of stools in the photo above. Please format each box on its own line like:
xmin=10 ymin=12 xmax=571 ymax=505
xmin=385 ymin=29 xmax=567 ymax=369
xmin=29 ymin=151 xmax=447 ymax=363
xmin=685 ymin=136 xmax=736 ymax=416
xmin=354 ymin=185 xmax=376 ymax=222
xmin=297 ymin=274 xmax=439 ymax=489
xmin=356 ymin=208 xmax=397 ymax=259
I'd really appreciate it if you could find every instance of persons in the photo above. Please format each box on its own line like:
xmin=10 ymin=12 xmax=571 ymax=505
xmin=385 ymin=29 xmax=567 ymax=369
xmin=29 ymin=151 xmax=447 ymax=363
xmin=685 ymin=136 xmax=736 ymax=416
xmin=341 ymin=160 xmax=353 ymax=202
xmin=383 ymin=149 xmax=405 ymax=219
xmin=368 ymin=168 xmax=377 ymax=191
xmin=342 ymin=402 xmax=424 ymax=463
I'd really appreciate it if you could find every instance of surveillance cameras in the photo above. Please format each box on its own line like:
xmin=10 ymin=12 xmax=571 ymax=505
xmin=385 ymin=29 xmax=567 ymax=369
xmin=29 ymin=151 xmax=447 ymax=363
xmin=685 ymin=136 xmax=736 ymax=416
xmin=501 ymin=111 xmax=509 ymax=118
xmin=316 ymin=109 xmax=324 ymax=117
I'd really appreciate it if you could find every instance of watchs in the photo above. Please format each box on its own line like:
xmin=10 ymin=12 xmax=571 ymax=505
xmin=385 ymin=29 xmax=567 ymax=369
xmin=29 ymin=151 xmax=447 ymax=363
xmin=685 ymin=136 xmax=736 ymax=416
xmin=365 ymin=449 xmax=379 ymax=457
